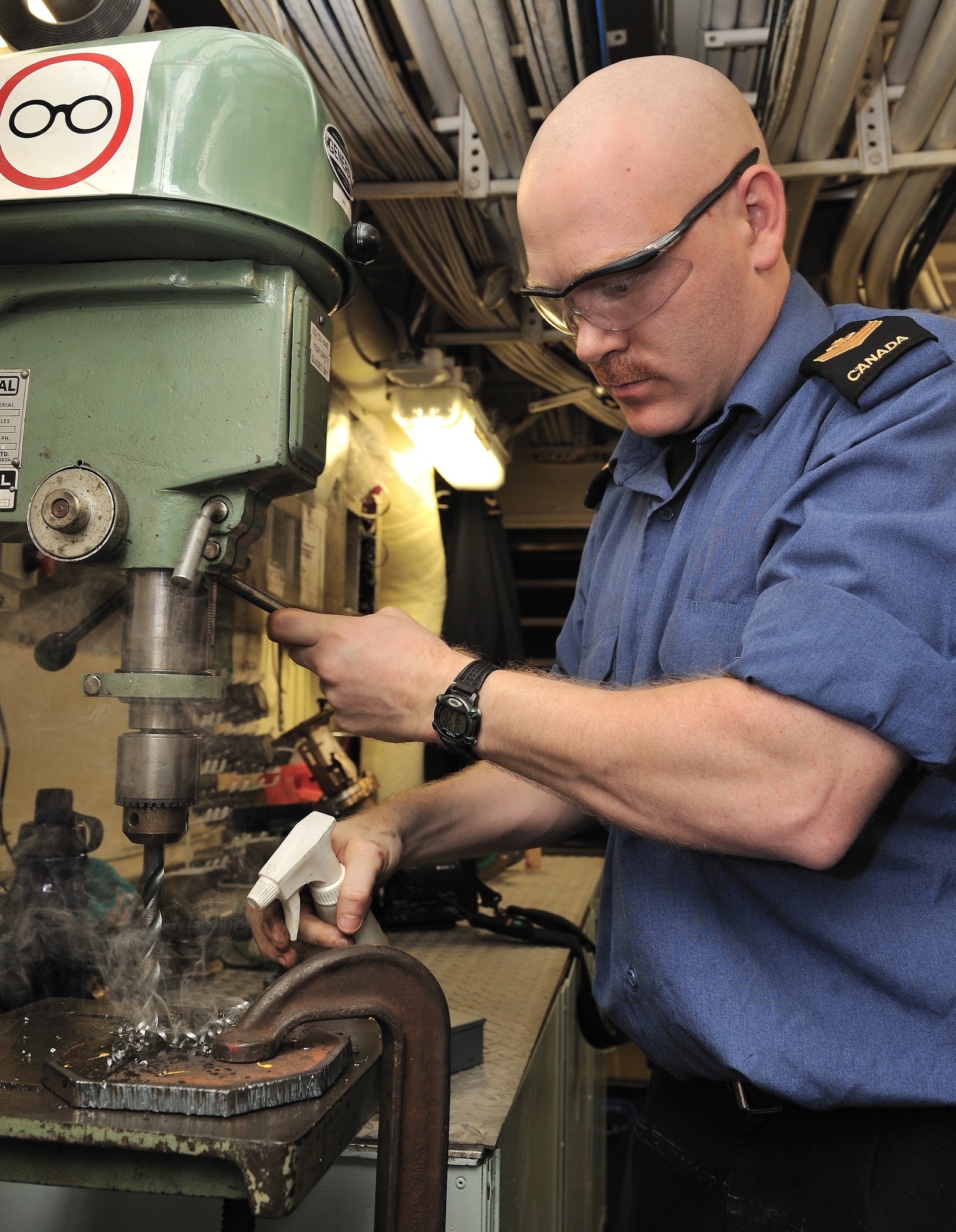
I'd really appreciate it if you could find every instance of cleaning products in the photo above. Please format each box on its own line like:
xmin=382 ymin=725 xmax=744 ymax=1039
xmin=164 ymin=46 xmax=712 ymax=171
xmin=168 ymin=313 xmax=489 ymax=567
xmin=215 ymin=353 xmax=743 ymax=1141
xmin=244 ymin=810 xmax=388 ymax=953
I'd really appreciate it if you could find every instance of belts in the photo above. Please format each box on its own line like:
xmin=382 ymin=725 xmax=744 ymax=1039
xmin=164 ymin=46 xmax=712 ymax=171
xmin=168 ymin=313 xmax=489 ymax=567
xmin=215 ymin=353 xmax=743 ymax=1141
xmin=649 ymin=1069 xmax=809 ymax=1122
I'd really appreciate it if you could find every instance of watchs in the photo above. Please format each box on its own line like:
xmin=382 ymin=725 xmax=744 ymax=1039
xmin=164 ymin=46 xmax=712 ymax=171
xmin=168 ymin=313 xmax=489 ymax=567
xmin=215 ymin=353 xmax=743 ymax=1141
xmin=431 ymin=660 xmax=500 ymax=759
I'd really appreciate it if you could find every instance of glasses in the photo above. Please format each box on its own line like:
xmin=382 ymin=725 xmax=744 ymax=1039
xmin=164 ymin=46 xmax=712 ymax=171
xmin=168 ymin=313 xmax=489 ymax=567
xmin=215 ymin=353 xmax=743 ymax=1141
xmin=520 ymin=147 xmax=760 ymax=339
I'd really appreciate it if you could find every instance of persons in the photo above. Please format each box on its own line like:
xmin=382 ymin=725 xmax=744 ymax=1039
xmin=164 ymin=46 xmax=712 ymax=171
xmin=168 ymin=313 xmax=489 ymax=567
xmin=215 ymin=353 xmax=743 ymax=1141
xmin=245 ymin=49 xmax=955 ymax=1229
